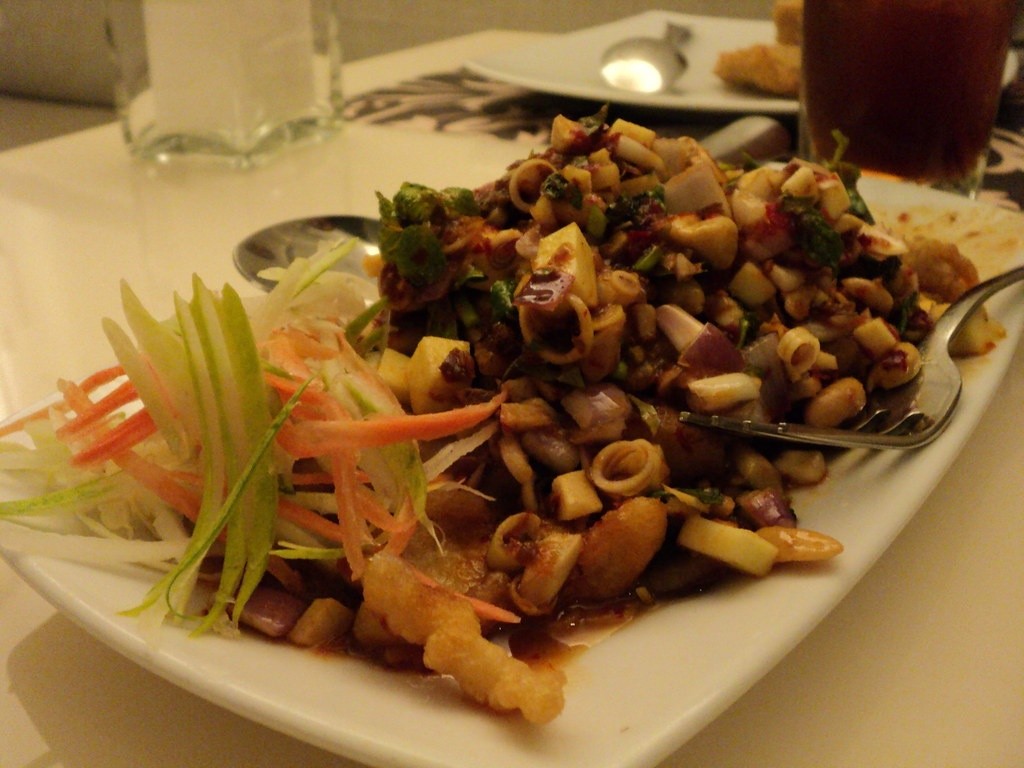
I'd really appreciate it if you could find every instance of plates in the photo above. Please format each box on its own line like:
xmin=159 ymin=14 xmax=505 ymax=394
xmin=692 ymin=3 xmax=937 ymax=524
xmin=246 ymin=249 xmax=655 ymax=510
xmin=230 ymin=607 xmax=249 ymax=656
xmin=2 ymin=181 xmax=1024 ymax=768
xmin=466 ymin=11 xmax=1020 ymax=119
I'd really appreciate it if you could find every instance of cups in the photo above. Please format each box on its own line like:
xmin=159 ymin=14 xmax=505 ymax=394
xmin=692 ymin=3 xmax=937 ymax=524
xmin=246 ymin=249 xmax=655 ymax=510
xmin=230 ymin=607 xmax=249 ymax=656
xmin=798 ymin=0 xmax=1016 ymax=191
xmin=102 ymin=0 xmax=344 ymax=176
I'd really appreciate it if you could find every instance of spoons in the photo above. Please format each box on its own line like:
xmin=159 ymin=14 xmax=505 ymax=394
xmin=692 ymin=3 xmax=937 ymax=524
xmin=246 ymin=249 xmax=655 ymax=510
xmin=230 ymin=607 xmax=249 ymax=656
xmin=601 ymin=20 xmax=687 ymax=95
xmin=232 ymin=213 xmax=388 ymax=293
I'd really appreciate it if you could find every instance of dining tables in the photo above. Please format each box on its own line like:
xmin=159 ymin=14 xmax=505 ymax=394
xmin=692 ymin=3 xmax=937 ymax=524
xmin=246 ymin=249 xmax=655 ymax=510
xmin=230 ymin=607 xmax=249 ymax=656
xmin=0 ymin=28 xmax=1024 ymax=767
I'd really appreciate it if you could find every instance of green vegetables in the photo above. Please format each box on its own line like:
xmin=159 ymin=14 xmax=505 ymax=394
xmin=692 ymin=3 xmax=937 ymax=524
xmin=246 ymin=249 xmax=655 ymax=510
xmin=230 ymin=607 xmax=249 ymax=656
xmin=542 ymin=127 xmax=875 ymax=264
xmin=376 ymin=177 xmax=514 ymax=320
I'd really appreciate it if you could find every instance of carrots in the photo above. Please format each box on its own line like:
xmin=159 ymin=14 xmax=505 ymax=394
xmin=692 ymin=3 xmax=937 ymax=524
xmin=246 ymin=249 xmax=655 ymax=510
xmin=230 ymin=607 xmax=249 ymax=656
xmin=0 ymin=331 xmax=519 ymax=627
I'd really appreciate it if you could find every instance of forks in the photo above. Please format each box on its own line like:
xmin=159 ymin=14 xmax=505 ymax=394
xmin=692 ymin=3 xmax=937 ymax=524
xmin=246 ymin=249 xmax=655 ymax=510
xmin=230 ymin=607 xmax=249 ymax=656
xmin=681 ymin=266 xmax=1021 ymax=445
